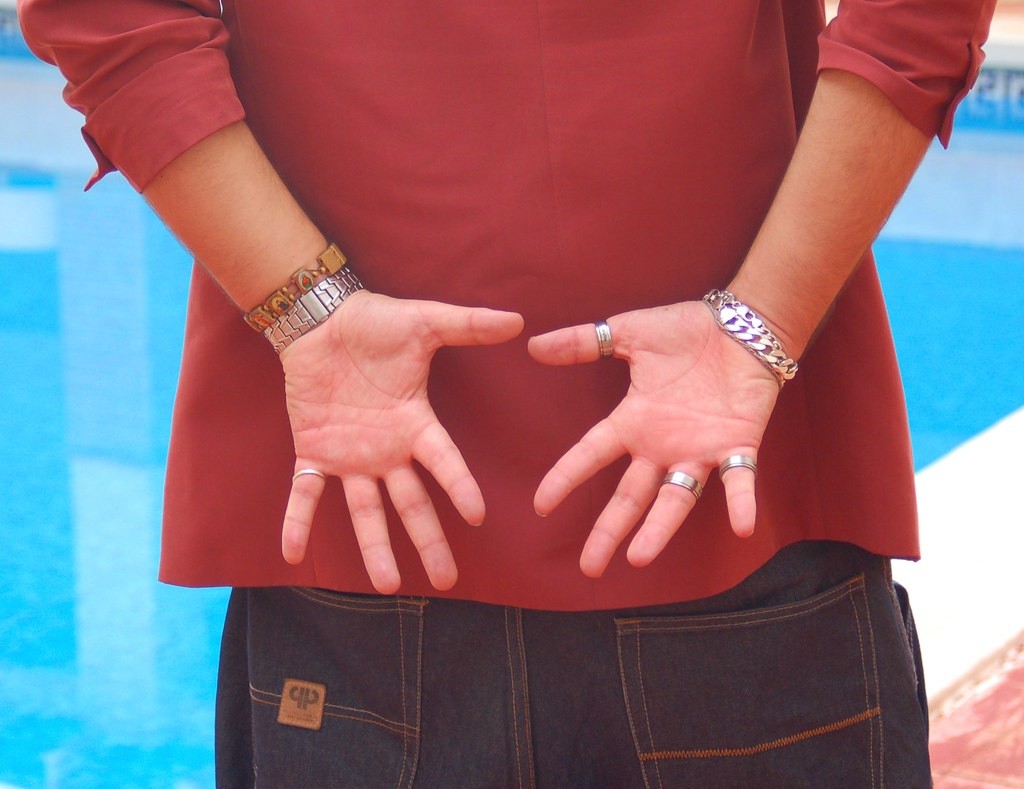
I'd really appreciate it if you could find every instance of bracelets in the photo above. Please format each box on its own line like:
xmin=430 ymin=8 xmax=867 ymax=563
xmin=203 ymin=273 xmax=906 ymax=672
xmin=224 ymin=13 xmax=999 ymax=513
xmin=243 ymin=239 xmax=348 ymax=332
xmin=262 ymin=263 xmax=365 ymax=355
xmin=700 ymin=284 xmax=800 ymax=387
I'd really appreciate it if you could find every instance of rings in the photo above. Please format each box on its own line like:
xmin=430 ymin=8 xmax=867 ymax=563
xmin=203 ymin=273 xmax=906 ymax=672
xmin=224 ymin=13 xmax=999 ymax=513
xmin=717 ymin=453 xmax=758 ymax=479
xmin=660 ymin=470 xmax=704 ymax=499
xmin=291 ymin=468 xmax=328 ymax=482
xmin=593 ymin=319 xmax=614 ymax=357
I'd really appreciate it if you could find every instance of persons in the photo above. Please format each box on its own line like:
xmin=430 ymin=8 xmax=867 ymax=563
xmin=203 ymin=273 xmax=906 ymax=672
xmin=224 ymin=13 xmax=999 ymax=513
xmin=14 ymin=1 xmax=995 ymax=786
